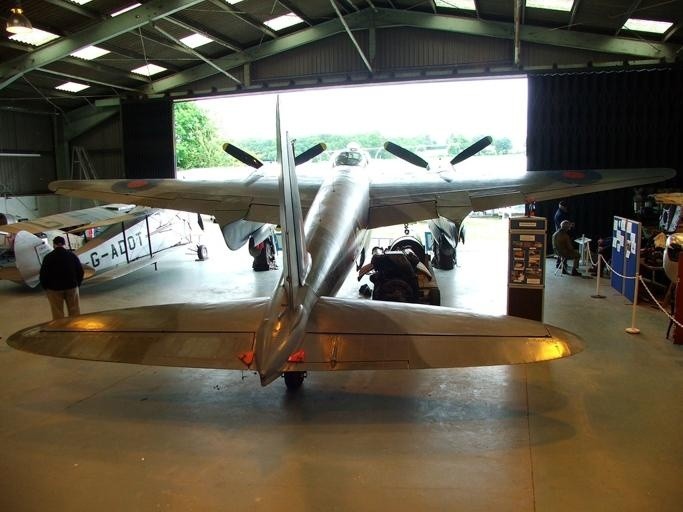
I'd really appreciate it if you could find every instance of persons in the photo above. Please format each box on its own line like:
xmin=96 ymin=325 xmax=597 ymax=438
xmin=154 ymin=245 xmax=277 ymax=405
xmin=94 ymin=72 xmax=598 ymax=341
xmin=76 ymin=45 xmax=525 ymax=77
xmin=39 ymin=235 xmax=84 ymax=322
xmin=551 ymin=201 xmax=575 ymax=269
xmin=551 ymin=218 xmax=582 ymax=276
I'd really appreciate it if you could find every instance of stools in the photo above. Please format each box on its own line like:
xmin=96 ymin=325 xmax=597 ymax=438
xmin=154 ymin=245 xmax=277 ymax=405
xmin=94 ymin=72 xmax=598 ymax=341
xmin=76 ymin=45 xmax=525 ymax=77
xmin=553 ymin=255 xmax=572 ymax=277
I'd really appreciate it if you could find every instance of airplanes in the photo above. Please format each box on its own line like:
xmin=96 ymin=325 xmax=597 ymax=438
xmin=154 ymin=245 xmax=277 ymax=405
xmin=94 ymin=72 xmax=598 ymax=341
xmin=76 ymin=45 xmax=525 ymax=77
xmin=0 ymin=203 xmax=214 ymax=288
xmin=5 ymin=93 xmax=676 ymax=390
